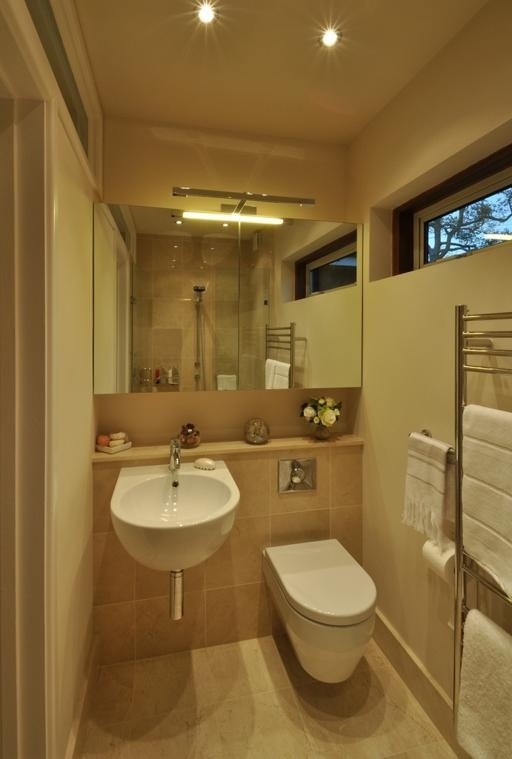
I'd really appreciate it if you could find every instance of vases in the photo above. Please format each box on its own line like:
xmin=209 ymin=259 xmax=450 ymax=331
xmin=315 ymin=427 xmax=330 ymax=441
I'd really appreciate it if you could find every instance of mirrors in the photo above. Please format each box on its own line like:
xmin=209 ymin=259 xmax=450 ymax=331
xmin=93 ymin=200 xmax=362 ymax=394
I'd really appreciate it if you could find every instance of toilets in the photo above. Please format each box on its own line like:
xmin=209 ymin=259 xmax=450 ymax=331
xmin=262 ymin=537 xmax=377 ymax=686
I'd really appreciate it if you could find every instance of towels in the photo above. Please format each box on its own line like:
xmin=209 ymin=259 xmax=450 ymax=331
xmin=460 ymin=403 xmax=512 ymax=601
xmin=401 ymin=431 xmax=456 ymax=555
xmin=264 ymin=359 xmax=290 ymax=390
xmin=217 ymin=375 xmax=237 ymax=391
xmin=456 ymin=609 xmax=511 ymax=759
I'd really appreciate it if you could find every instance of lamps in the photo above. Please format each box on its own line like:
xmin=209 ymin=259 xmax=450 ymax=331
xmin=170 ymin=210 xmax=293 ymax=226
xmin=173 ymin=186 xmax=315 ymax=207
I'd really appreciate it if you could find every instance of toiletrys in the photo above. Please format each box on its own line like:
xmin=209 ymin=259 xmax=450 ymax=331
xmin=154 ymin=368 xmax=161 ymax=384
xmin=166 ymin=369 xmax=174 ymax=384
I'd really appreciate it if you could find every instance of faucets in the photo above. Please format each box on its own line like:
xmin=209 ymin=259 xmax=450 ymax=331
xmin=168 ymin=439 xmax=181 ymax=472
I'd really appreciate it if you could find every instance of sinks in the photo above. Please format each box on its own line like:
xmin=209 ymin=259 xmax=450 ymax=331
xmin=110 ymin=460 xmax=240 ymax=571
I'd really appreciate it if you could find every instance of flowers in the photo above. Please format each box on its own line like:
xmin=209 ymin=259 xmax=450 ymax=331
xmin=299 ymin=396 xmax=342 ymax=428
